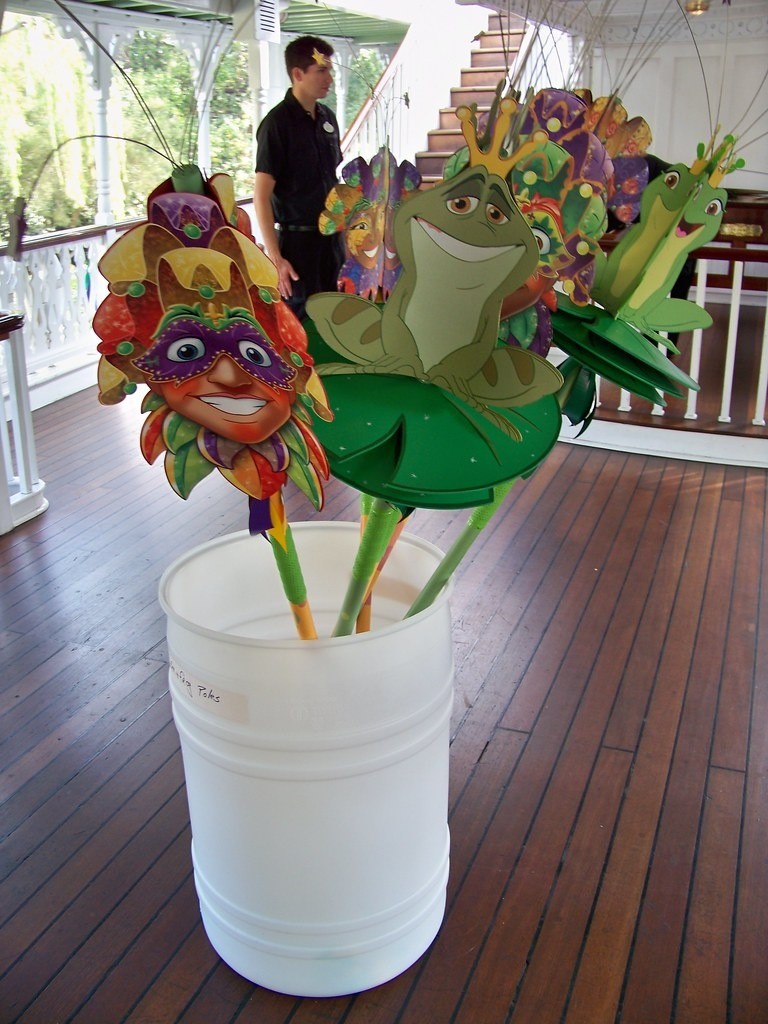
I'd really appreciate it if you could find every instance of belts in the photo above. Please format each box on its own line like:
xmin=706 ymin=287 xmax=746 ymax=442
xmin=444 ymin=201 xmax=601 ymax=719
xmin=273 ymin=223 xmax=317 ymax=232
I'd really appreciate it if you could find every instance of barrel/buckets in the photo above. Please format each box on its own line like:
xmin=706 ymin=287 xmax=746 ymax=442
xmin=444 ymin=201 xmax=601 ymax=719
xmin=158 ymin=520 xmax=451 ymax=997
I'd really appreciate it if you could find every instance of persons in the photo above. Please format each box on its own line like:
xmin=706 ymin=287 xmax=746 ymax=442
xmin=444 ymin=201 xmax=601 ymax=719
xmin=255 ymin=36 xmax=344 ymax=320
